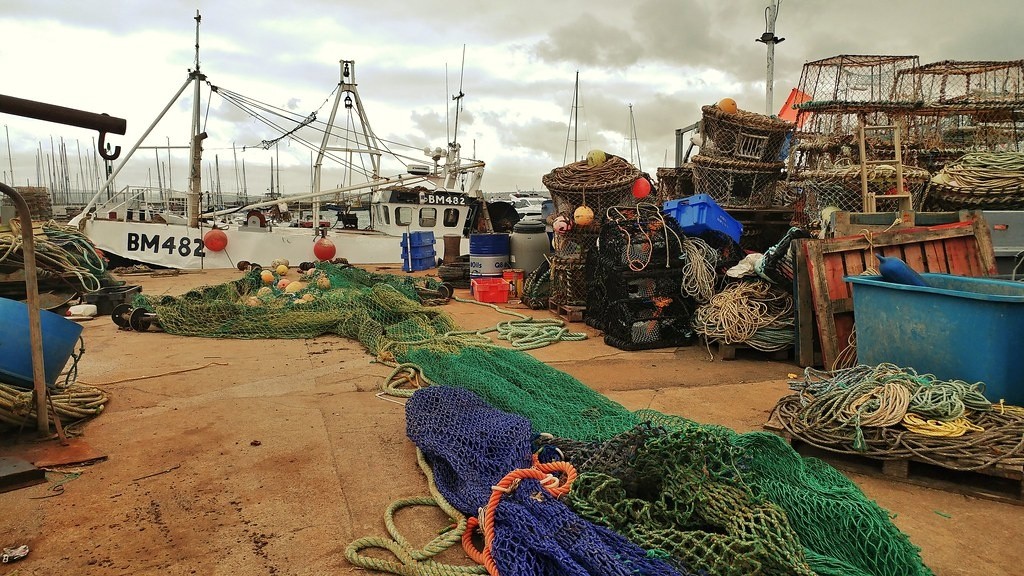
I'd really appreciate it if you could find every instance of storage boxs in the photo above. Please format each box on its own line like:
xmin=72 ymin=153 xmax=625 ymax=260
xmin=400 ymin=230 xmax=436 ymax=247
xmin=402 ymin=256 xmax=436 ymax=271
xmin=401 ymin=244 xmax=436 ymax=259
xmin=664 ymin=193 xmax=744 ymax=245
xmin=82 ymin=285 xmax=142 ymax=316
xmin=471 ymin=278 xmax=510 ymax=303
xmin=842 ymin=272 xmax=1024 ymax=409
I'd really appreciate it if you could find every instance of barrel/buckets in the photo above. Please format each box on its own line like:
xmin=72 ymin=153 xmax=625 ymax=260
xmin=470 ymin=233 xmax=510 ymax=296
xmin=502 ymin=269 xmax=525 ymax=299
xmin=510 ymin=220 xmax=550 ymax=280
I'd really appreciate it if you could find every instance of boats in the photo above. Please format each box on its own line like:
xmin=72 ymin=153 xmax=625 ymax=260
xmin=486 ymin=186 xmax=553 ymax=224
xmin=66 ymin=8 xmax=486 ymax=266
xmin=25 ymin=133 xmax=377 ymax=226
